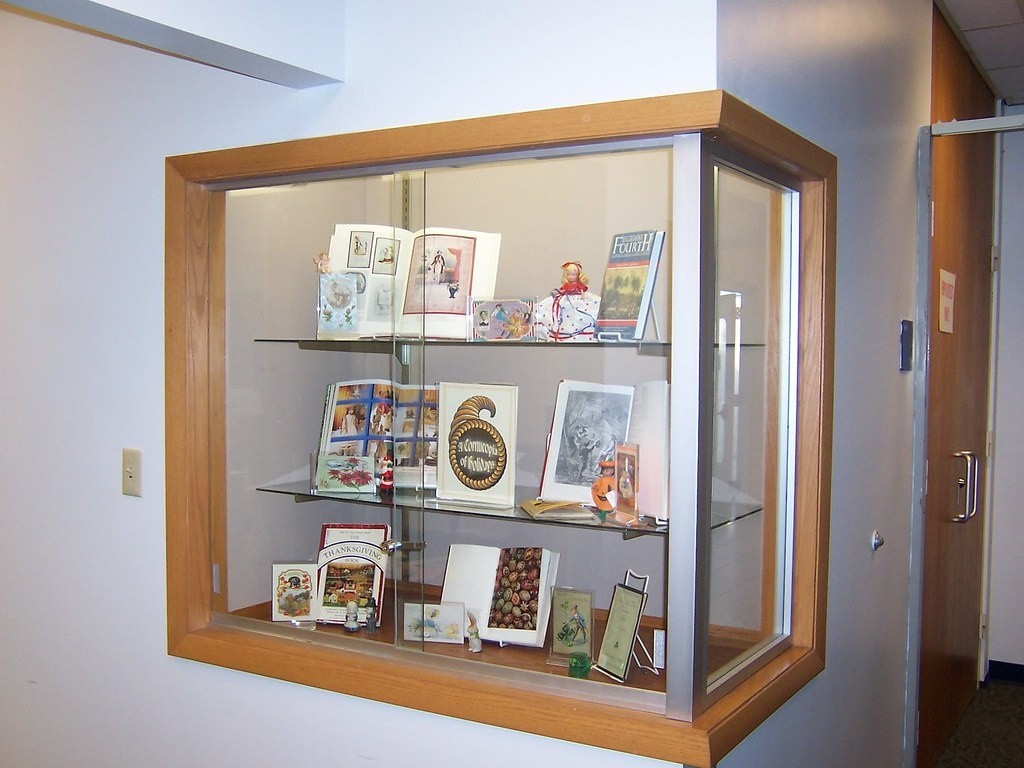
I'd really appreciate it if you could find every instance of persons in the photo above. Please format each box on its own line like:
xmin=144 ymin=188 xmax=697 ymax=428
xmin=534 ymin=262 xmax=603 ymax=342
xmin=344 ymin=601 xmax=360 ymax=632
xmin=379 ymin=456 xmax=395 ymax=495
xmin=592 ymin=461 xmax=617 ymax=523
xmin=365 ymin=596 xmax=378 ymax=635
xmin=479 ymin=311 xmax=489 ymax=327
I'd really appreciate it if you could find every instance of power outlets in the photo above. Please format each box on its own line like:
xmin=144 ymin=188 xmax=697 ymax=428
xmin=122 ymin=447 xmax=143 ymax=498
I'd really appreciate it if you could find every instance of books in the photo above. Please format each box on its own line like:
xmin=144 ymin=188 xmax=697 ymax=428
xmin=590 ymin=230 xmax=669 ymax=340
xmin=309 ymin=522 xmax=394 ymax=627
xmin=440 ymin=543 xmax=561 ymax=648
xmin=317 ymin=224 xmax=502 ymax=342
xmin=315 ymin=379 xmax=439 ymax=492
xmin=272 ymin=561 xmax=317 ymax=622
xmin=521 ymin=500 xmax=600 ymax=527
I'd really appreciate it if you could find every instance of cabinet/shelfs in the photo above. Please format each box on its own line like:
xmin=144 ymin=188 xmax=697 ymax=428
xmin=166 ymin=89 xmax=838 ymax=768
